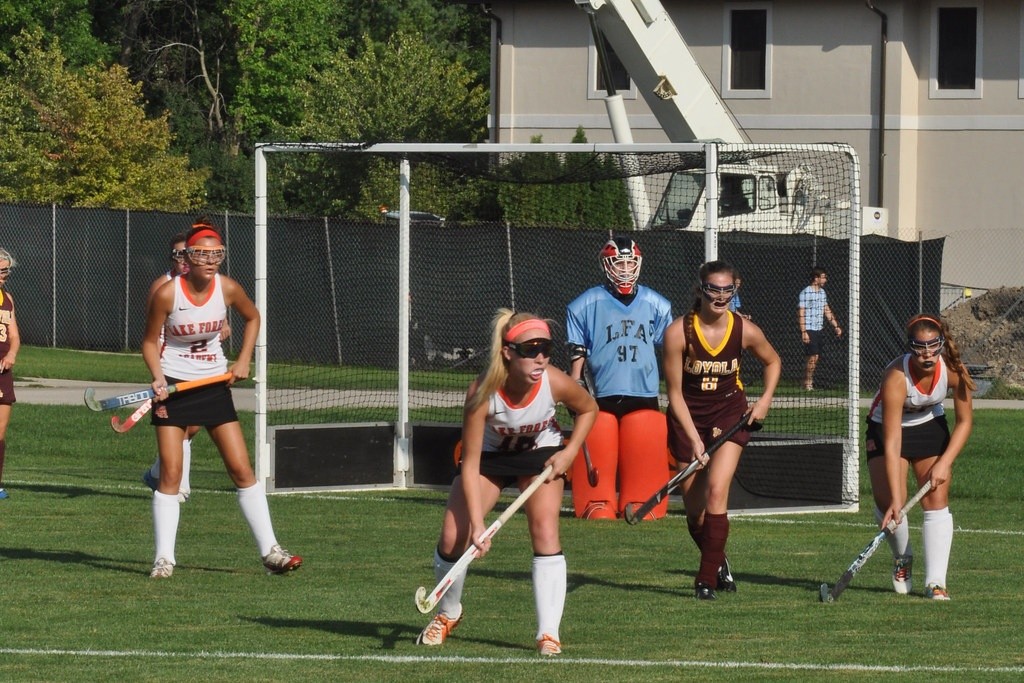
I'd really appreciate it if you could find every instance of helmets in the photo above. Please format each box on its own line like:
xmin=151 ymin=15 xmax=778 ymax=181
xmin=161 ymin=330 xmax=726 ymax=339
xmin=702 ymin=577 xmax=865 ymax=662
xmin=599 ymin=236 xmax=643 ymax=296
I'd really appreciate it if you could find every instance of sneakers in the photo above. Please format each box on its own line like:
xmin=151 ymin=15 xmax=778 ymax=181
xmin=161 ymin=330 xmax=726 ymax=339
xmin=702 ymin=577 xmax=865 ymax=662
xmin=925 ymin=583 xmax=951 ymax=601
xmin=143 ymin=469 xmax=160 ymax=493
xmin=416 ymin=601 xmax=463 ymax=646
xmin=177 ymin=488 xmax=190 ymax=504
xmin=891 ymin=553 xmax=913 ymax=594
xmin=716 ymin=552 xmax=736 ymax=594
xmin=261 ymin=544 xmax=302 ymax=575
xmin=535 ymin=634 xmax=562 ymax=656
xmin=0 ymin=488 xmax=8 ymax=499
xmin=149 ymin=556 xmax=174 ymax=578
xmin=694 ymin=582 xmax=717 ymax=603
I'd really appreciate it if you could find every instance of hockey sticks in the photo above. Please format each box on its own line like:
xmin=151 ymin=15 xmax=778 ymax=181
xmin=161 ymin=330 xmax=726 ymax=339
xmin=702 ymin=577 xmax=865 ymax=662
xmin=415 ymin=462 xmax=556 ymax=615
xmin=818 ymin=478 xmax=932 ymax=605
xmin=624 ymin=411 xmax=753 ymax=527
xmin=109 ymin=397 xmax=152 ymax=435
xmin=83 ymin=370 xmax=252 ymax=413
xmin=564 ymin=370 xmax=600 ymax=488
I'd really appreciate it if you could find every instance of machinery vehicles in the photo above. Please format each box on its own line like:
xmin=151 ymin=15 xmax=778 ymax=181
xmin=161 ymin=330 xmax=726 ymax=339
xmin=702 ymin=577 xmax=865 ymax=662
xmin=573 ymin=0 xmax=887 ymax=238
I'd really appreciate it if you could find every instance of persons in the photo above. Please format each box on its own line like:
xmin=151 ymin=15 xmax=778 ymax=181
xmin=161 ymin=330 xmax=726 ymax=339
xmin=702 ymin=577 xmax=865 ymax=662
xmin=144 ymin=234 xmax=231 ymax=503
xmin=663 ymin=262 xmax=781 ymax=600
xmin=865 ymin=314 xmax=977 ymax=599
xmin=798 ymin=267 xmax=841 ymax=391
xmin=415 ymin=308 xmax=600 ymax=655
xmin=727 ymin=275 xmax=753 ymax=386
xmin=142 ymin=219 xmax=303 ymax=578
xmin=566 ymin=238 xmax=673 ymax=521
xmin=0 ymin=246 xmax=20 ymax=500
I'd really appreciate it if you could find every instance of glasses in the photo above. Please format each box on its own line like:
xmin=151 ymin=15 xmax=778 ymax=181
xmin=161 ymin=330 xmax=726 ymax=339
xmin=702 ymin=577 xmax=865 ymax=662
xmin=501 ymin=339 xmax=555 ymax=358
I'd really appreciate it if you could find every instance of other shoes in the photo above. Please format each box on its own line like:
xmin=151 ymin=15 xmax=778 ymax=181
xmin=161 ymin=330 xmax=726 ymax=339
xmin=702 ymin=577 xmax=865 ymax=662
xmin=800 ymin=382 xmax=814 ymax=391
